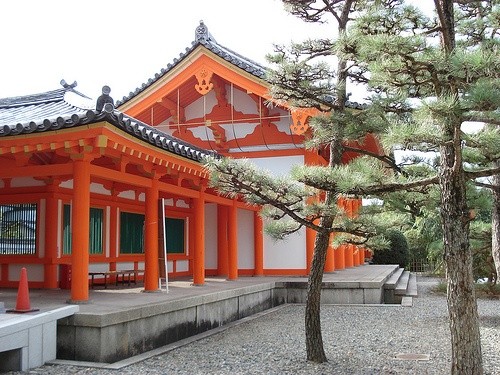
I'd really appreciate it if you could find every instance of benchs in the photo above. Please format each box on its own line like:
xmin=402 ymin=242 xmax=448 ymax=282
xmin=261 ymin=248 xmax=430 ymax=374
xmin=88 ymin=270 xmax=146 ymax=288
